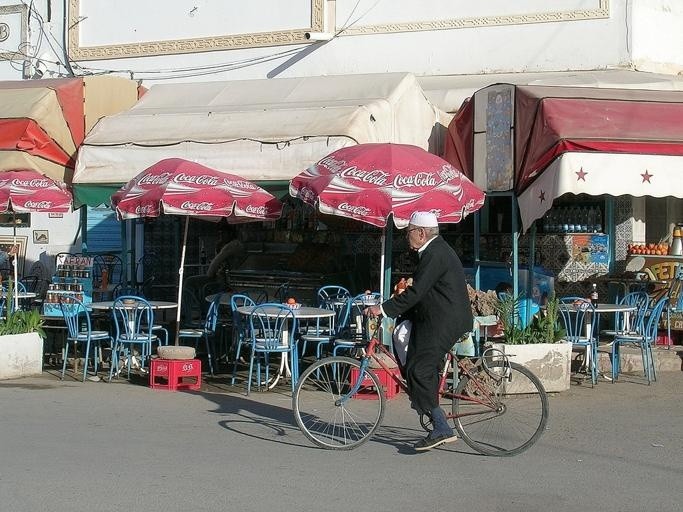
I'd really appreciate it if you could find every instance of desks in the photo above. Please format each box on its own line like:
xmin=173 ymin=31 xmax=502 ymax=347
xmin=540 ymin=302 xmax=637 ymax=315
xmin=83 ymin=297 xmax=177 ymax=314
xmin=236 ymin=303 xmax=337 ymax=319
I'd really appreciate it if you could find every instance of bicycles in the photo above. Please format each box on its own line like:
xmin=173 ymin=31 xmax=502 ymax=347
xmin=290 ymin=312 xmax=551 ymax=459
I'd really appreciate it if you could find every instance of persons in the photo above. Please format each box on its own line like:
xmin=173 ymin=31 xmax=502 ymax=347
xmin=362 ymin=210 xmax=474 ymax=450
xmin=183 ymin=224 xmax=242 ymax=302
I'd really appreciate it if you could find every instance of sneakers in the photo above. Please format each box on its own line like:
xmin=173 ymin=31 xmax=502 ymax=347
xmin=414 ymin=430 xmax=458 ymax=451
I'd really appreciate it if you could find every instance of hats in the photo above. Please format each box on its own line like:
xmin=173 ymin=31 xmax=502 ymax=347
xmin=409 ymin=211 xmax=439 ymax=228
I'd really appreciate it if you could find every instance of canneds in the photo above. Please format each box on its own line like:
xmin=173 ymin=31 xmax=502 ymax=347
xmin=46 ymin=263 xmax=90 ymax=303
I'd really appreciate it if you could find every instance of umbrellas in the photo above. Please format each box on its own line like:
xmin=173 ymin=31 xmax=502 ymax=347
xmin=288 ymin=142 xmax=485 ymax=354
xmin=111 ymin=158 xmax=283 ymax=346
xmin=0 ymin=169 xmax=73 ymax=312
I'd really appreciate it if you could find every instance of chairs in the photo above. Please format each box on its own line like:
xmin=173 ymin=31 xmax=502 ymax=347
xmin=109 ymin=297 xmax=157 ymax=381
xmin=603 ymin=291 xmax=649 ymax=338
xmin=612 ymin=297 xmax=670 ymax=383
xmin=53 ymin=294 xmax=112 ymax=380
xmin=244 ymin=303 xmax=299 ymax=395
xmin=138 ymin=324 xmax=168 ymax=347
xmin=300 ymin=285 xmax=352 ymax=384
xmin=554 ymin=297 xmax=601 ymax=390
xmin=229 ymin=295 xmax=258 ymax=381
xmin=332 ymin=291 xmax=382 ymax=382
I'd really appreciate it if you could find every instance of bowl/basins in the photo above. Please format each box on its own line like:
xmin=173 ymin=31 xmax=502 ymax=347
xmin=281 ymin=302 xmax=301 ymax=310
xmin=122 ymin=299 xmax=134 ymax=304
xmin=574 ymin=303 xmax=590 ymax=311
xmin=361 ymin=295 xmax=375 ymax=300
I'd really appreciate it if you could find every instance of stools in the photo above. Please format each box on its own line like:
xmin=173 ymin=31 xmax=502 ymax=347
xmin=151 ymin=356 xmax=203 ymax=390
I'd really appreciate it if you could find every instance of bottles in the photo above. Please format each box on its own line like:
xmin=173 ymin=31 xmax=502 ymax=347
xmin=590 ymin=283 xmax=599 ymax=309
xmin=0 ymin=272 xmax=2 ymax=297
xmin=396 ymin=278 xmax=406 ymax=295
xmin=47 ymin=265 xmax=88 ymax=303
xmin=101 ymin=267 xmax=107 ymax=289
xmin=543 ymin=204 xmax=603 ymax=234
xmin=540 ymin=290 xmax=548 ymax=305
